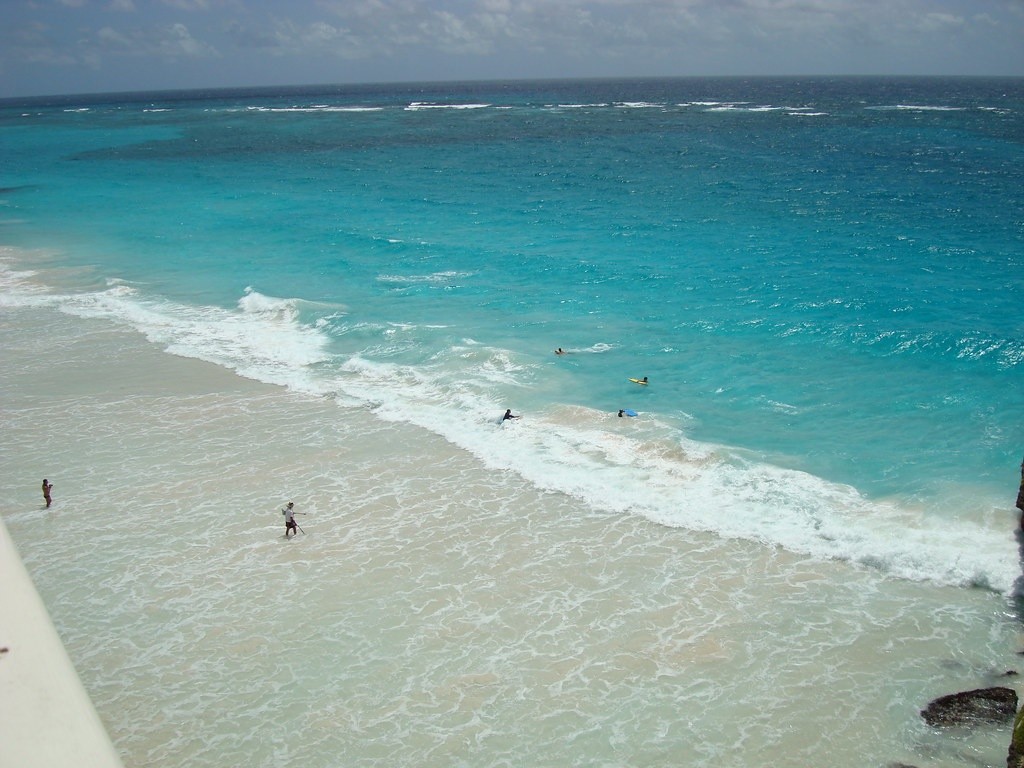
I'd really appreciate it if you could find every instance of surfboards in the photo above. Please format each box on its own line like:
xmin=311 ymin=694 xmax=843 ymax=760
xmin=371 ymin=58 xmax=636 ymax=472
xmin=629 ymin=377 xmax=650 ymax=385
xmin=623 ymin=408 xmax=639 ymax=417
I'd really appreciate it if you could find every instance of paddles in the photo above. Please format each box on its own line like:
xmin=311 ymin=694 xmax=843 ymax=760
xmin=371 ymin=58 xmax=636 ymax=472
xmin=281 ymin=509 xmax=307 ymax=515
xmin=297 ymin=524 xmax=306 ymax=536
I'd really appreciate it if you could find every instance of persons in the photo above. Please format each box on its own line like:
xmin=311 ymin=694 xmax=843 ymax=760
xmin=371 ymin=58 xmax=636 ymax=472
xmin=618 ymin=409 xmax=625 ymax=417
xmin=286 ymin=501 xmax=297 ymax=535
xmin=42 ymin=479 xmax=53 ymax=507
xmin=640 ymin=376 xmax=648 ymax=382
xmin=503 ymin=409 xmax=515 ymax=421
xmin=555 ymin=348 xmax=564 ymax=354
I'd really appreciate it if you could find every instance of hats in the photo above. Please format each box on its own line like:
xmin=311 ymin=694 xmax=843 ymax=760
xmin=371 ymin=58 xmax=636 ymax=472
xmin=286 ymin=501 xmax=294 ymax=508
